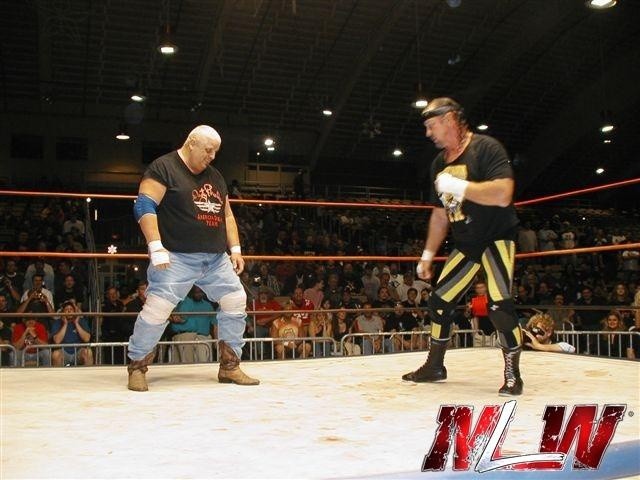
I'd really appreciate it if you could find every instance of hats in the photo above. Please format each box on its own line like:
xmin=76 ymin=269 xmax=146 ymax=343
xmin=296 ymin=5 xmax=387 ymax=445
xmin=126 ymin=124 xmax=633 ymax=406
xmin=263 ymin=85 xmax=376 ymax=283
xmin=257 ymin=285 xmax=269 ymax=294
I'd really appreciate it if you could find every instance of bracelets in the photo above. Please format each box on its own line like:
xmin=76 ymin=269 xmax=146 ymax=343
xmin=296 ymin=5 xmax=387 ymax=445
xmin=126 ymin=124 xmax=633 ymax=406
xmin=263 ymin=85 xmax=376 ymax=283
xmin=146 ymin=239 xmax=164 ymax=252
xmin=229 ymin=245 xmax=242 ymax=254
xmin=420 ymin=249 xmax=435 ymax=262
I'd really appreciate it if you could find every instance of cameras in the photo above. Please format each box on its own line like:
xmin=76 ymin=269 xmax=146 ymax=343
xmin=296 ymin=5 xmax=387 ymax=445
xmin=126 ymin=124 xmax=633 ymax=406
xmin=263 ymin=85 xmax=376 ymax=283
xmin=522 ymin=326 xmax=544 ymax=350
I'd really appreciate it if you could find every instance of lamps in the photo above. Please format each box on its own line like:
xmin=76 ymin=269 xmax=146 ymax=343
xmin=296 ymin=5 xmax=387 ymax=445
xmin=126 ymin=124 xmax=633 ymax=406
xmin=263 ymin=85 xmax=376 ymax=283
xmin=155 ymin=0 xmax=181 ymax=57
xmin=410 ymin=1 xmax=431 ymax=109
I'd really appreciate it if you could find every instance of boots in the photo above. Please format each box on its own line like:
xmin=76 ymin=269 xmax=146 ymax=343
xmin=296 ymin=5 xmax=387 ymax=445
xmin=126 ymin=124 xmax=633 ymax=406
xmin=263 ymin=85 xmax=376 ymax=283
xmin=497 ymin=340 xmax=524 ymax=398
xmin=401 ymin=335 xmax=452 ymax=383
xmin=217 ymin=339 xmax=260 ymax=386
xmin=128 ymin=349 xmax=155 ymax=393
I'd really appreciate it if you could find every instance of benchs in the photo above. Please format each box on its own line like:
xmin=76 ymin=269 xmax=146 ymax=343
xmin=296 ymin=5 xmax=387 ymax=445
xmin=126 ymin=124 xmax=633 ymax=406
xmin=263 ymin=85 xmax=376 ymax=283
xmin=306 ymin=190 xmax=639 ymax=261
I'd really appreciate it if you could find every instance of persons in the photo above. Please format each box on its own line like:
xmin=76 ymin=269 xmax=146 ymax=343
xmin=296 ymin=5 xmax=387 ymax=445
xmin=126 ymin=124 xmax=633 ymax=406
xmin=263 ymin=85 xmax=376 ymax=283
xmin=399 ymin=95 xmax=526 ymax=398
xmin=125 ymin=122 xmax=262 ymax=393
